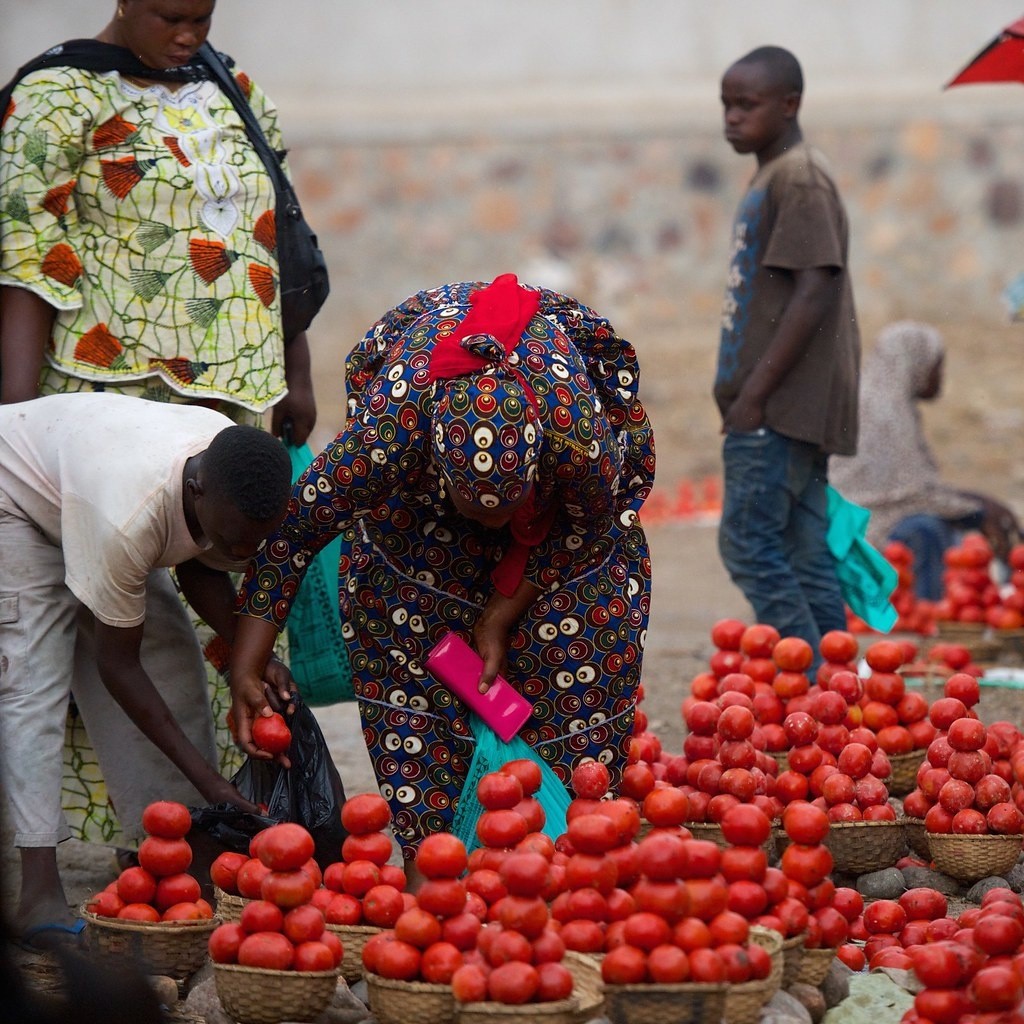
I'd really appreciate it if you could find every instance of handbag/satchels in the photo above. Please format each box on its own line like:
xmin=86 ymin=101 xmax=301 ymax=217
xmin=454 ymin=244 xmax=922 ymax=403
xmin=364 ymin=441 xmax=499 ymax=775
xmin=282 ymin=440 xmax=355 ymax=707
xmin=183 ymin=696 xmax=351 ymax=876
xmin=450 ymin=710 xmax=572 ymax=878
xmin=271 ymin=185 xmax=331 ymax=331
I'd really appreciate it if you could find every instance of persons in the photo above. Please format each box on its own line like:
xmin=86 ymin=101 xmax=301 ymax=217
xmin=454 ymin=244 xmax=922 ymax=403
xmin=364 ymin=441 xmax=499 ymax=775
xmin=0 ymin=0 xmax=318 ymax=875
xmin=231 ymin=274 xmax=656 ymax=864
xmin=828 ymin=322 xmax=1022 ymax=602
xmin=711 ymin=46 xmax=861 ymax=688
xmin=0 ymin=392 xmax=298 ymax=926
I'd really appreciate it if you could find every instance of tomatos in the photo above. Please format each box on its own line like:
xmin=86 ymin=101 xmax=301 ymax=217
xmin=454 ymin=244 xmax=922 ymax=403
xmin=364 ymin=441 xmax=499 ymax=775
xmin=84 ymin=618 xmax=1024 ymax=1024
xmin=844 ymin=533 xmax=1024 ymax=636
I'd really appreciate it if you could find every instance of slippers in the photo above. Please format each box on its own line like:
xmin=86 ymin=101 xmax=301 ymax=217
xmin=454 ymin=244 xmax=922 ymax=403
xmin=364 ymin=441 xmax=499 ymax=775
xmin=13 ymin=912 xmax=87 ymax=960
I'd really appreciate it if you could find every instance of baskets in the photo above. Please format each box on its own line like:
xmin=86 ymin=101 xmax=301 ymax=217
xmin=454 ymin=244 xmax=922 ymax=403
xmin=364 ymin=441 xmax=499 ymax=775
xmin=850 ymin=613 xmax=1024 ymax=662
xmin=79 ymin=749 xmax=1024 ymax=1024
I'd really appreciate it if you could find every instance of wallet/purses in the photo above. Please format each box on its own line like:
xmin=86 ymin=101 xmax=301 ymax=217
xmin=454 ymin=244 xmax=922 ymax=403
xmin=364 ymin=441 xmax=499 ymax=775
xmin=422 ymin=631 xmax=534 ymax=744
